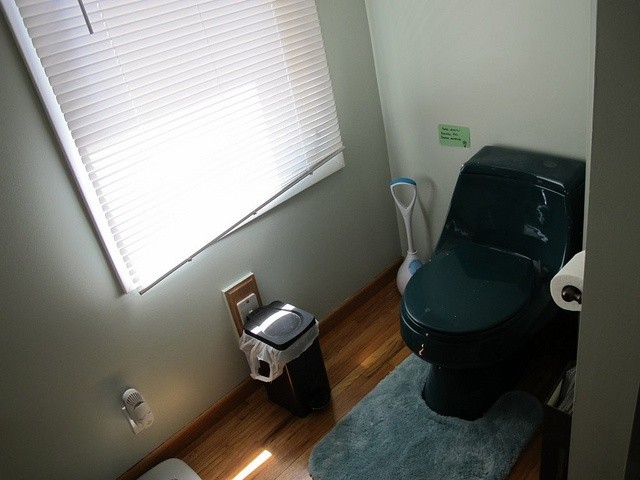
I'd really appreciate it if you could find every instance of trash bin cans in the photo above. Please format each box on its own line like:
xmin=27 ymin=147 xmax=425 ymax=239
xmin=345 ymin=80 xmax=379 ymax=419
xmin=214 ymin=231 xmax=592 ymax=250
xmin=242 ymin=301 xmax=331 ymax=419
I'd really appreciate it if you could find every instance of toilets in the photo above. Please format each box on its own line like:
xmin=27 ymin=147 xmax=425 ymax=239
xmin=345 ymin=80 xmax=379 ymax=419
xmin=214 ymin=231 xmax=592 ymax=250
xmin=394 ymin=144 xmax=586 ymax=423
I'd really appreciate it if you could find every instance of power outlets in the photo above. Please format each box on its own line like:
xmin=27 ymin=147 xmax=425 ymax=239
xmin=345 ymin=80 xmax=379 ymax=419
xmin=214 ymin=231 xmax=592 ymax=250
xmin=122 ymin=405 xmax=147 ymax=434
